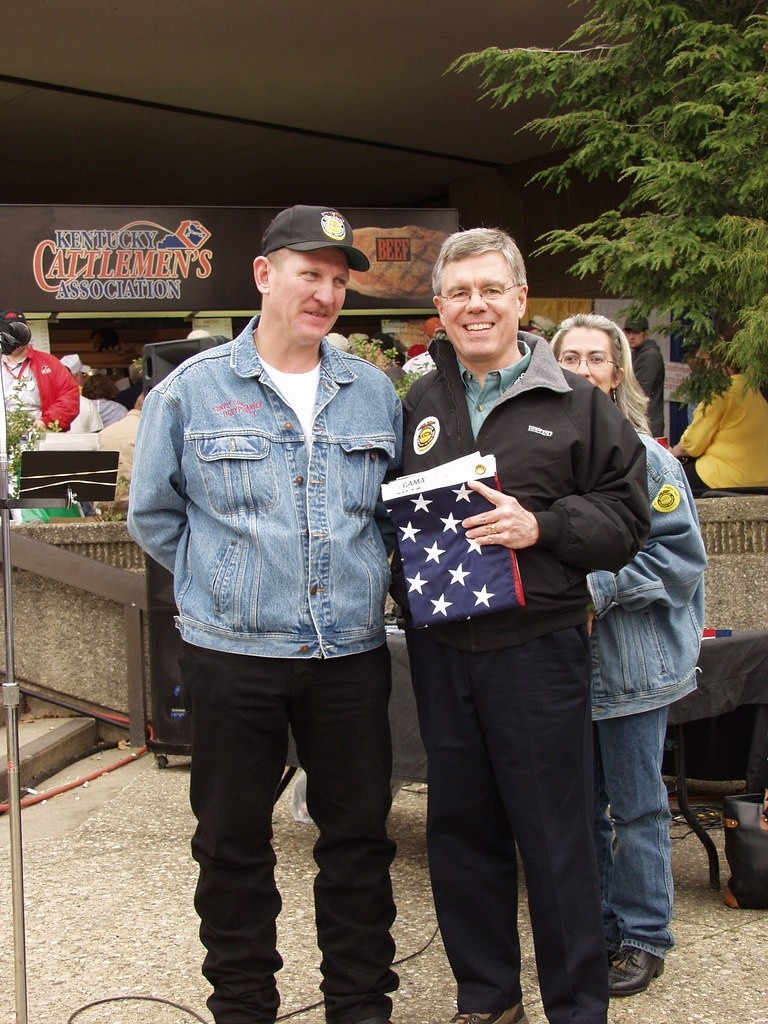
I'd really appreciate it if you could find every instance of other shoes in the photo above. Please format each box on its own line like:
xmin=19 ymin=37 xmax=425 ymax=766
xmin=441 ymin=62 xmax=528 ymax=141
xmin=449 ymin=1001 xmax=529 ymax=1024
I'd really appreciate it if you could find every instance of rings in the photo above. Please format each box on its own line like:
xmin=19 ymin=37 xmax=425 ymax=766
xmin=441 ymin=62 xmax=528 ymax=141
xmin=491 ymin=524 xmax=498 ymax=533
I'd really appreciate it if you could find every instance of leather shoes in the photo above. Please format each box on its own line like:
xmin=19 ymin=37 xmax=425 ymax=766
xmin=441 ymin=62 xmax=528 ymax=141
xmin=608 ymin=946 xmax=664 ymax=997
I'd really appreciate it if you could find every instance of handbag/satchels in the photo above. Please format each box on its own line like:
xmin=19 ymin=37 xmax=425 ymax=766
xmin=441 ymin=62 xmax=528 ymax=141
xmin=723 ymin=788 xmax=768 ymax=909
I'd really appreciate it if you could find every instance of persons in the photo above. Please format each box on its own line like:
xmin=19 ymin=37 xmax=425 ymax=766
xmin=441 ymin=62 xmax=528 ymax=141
xmin=372 ymin=228 xmax=649 ymax=1023
xmin=407 ymin=343 xmax=427 ymax=362
xmin=82 ymin=373 xmax=128 ymax=428
xmin=0 ymin=311 xmax=80 ymax=433
xmin=670 ymin=366 xmax=768 ymax=498
xmin=551 ymin=315 xmax=707 ymax=997
xmin=326 ymin=333 xmax=352 ymax=353
xmin=60 ymin=354 xmax=104 ymax=517
xmin=402 ymin=317 xmax=446 ymax=380
xmin=93 ymin=393 xmax=143 ymax=515
xmin=114 ymin=359 xmax=143 ymax=409
xmin=127 ymin=204 xmax=403 ymax=1024
xmin=623 ymin=313 xmax=665 ymax=437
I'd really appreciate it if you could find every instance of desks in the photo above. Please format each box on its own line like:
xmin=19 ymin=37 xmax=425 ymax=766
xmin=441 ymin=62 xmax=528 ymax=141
xmin=276 ymin=630 xmax=768 ymax=893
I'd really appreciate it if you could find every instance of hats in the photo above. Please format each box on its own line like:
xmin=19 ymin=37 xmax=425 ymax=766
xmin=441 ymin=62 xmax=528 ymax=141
xmin=61 ymin=354 xmax=91 ymax=374
xmin=261 ymin=205 xmax=370 ymax=272
xmin=0 ymin=308 xmax=25 ymax=324
xmin=623 ymin=314 xmax=649 ymax=333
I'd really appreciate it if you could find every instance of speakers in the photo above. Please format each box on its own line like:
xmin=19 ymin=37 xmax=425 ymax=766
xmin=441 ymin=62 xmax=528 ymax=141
xmin=143 ymin=337 xmax=234 ymax=755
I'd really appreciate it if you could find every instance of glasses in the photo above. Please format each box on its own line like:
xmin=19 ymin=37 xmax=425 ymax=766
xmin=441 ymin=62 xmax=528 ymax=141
xmin=557 ymin=354 xmax=619 ymax=370
xmin=440 ymin=283 xmax=521 ymax=307
